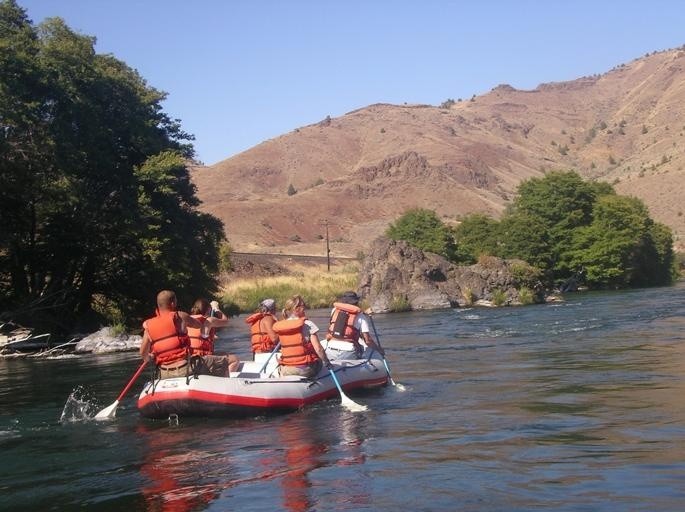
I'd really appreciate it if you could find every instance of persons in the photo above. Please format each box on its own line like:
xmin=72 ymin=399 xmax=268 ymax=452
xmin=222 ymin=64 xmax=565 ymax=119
xmin=244 ymin=297 xmax=284 ymax=356
xmin=136 ymin=288 xmax=208 ymax=379
xmin=184 ymin=298 xmax=241 ymax=378
xmin=267 ymin=292 xmax=335 ymax=380
xmin=321 ymin=288 xmax=381 ymax=363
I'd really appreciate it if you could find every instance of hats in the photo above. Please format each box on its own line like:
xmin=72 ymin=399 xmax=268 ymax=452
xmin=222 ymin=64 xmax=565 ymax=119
xmin=339 ymin=291 xmax=360 ymax=304
xmin=262 ymin=298 xmax=276 ymax=310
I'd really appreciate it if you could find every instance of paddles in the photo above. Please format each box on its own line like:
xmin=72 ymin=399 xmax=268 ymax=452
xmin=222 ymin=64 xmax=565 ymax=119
xmin=95 ymin=355 xmax=150 ymax=418
xmin=328 ymin=365 xmax=365 ymax=407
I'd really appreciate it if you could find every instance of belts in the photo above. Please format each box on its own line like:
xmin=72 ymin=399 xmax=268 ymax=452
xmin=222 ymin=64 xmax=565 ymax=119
xmin=159 ymin=361 xmax=188 ymax=371
xmin=327 ymin=347 xmax=355 ymax=353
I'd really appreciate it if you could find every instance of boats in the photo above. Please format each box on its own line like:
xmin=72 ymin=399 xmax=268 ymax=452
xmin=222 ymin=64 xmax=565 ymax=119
xmin=136 ymin=337 xmax=390 ymax=419
